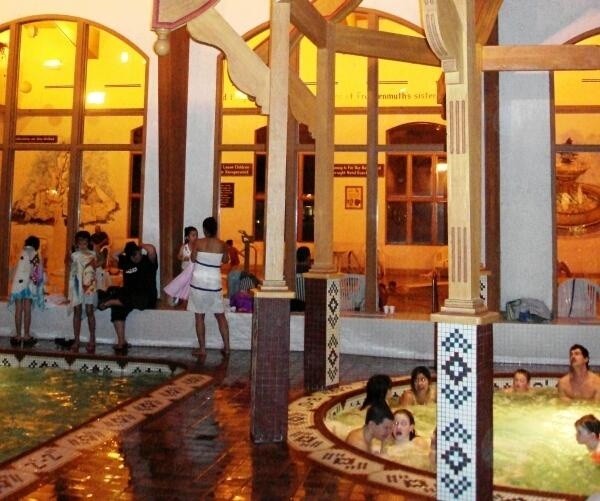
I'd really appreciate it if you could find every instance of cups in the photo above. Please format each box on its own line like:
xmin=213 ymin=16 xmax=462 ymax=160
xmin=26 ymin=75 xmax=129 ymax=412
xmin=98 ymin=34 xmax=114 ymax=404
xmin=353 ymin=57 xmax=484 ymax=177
xmin=383 ymin=306 xmax=395 ymax=316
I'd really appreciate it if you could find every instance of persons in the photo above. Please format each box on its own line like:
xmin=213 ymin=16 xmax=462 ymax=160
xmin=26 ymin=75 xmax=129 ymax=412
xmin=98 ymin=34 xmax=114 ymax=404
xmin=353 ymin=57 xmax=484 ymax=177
xmin=346 ymin=342 xmax=600 ymax=468
xmin=11 ymin=236 xmax=41 ymax=341
xmin=66 ymin=225 xmax=110 ymax=353
xmin=99 ymin=242 xmax=159 ymax=353
xmin=177 ymin=217 xmax=253 ymax=355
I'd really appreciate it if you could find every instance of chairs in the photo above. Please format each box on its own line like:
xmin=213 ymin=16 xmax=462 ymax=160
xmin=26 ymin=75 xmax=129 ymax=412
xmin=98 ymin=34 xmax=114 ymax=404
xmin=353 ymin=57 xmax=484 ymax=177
xmin=557 ymin=279 xmax=600 ymax=318
xmin=228 ymin=271 xmax=380 ymax=312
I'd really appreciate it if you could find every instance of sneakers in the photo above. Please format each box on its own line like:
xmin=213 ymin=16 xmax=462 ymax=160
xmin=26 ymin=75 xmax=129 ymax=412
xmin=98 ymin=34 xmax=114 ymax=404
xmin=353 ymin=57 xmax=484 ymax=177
xmin=9 ymin=335 xmax=38 ymax=346
xmin=68 ymin=341 xmax=96 ymax=352
xmin=221 ymin=348 xmax=231 ymax=357
xmin=112 ymin=342 xmax=133 ymax=353
xmin=192 ymin=348 xmax=208 ymax=357
